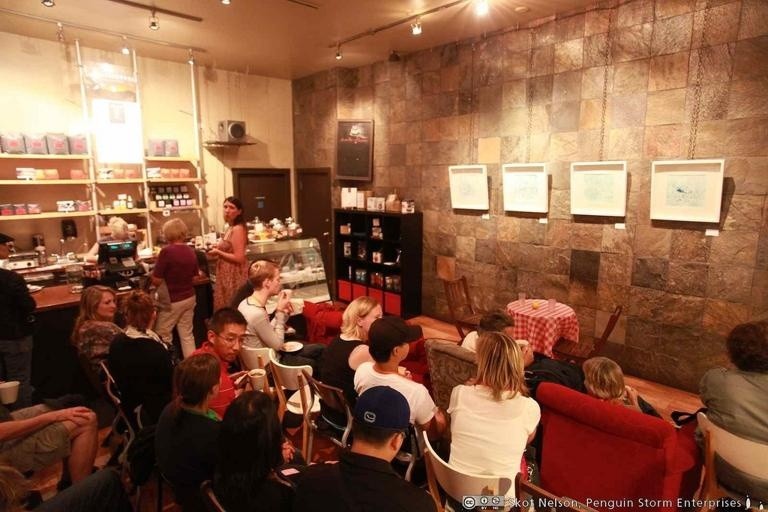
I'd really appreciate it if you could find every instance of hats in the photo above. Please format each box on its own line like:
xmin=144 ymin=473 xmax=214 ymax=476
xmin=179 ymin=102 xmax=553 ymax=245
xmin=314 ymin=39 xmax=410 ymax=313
xmin=352 ymin=384 xmax=411 ymax=432
xmin=367 ymin=315 xmax=423 ymax=346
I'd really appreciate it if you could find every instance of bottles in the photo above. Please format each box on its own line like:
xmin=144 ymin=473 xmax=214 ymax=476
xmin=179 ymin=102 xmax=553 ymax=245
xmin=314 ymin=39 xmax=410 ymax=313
xmin=57 ymin=235 xmax=89 ymax=256
xmin=246 ymin=215 xmax=303 ymax=245
xmin=128 ymin=194 xmax=134 ymax=209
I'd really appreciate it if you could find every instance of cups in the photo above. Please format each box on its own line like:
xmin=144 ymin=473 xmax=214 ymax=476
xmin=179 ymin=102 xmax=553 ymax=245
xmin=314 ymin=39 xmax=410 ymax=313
xmin=548 ymin=300 xmax=558 ymax=311
xmin=400 ymin=199 xmax=416 ymax=214
xmin=0 ymin=380 xmax=20 ymax=407
xmin=517 ymin=291 xmax=526 ymax=305
xmin=280 ymin=287 xmax=293 ymax=303
xmin=515 ymin=339 xmax=530 ymax=350
xmin=65 ymin=263 xmax=84 ymax=294
xmin=248 ymin=367 xmax=268 ymax=391
xmin=32 ymin=232 xmax=47 ymax=264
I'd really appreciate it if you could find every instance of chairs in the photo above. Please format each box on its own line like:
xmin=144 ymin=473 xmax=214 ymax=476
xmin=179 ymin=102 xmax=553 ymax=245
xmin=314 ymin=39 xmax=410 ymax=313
xmin=1 ymin=274 xmax=767 ymax=510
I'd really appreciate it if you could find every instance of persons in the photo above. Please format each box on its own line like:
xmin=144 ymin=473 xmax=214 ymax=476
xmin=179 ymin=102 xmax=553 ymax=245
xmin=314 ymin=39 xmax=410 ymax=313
xmin=583 ymin=357 xmax=665 ymax=421
xmin=206 ymin=195 xmax=252 ymax=313
xmin=0 ymin=381 xmax=99 ymax=483
xmin=71 ymin=261 xmax=583 ymax=512
xmin=1 ymin=469 xmax=133 ymax=511
xmin=150 ymin=219 xmax=200 ymax=359
xmin=86 ymin=216 xmax=149 ymax=264
xmin=693 ymin=317 xmax=768 ymax=512
xmin=0 ymin=232 xmax=38 ymax=399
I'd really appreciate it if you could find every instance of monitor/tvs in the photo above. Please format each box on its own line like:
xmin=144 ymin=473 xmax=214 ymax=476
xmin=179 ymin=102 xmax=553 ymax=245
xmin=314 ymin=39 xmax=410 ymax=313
xmin=97 ymin=240 xmax=136 ymax=266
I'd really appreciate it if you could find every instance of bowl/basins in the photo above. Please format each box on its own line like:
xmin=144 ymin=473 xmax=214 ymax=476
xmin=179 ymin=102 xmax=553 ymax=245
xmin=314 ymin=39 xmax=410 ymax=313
xmin=530 ymin=301 xmax=539 ymax=310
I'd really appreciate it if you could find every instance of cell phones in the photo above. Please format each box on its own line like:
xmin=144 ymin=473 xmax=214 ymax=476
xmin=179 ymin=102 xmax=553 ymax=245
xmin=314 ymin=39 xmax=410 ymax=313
xmin=279 ymin=466 xmax=301 ymax=479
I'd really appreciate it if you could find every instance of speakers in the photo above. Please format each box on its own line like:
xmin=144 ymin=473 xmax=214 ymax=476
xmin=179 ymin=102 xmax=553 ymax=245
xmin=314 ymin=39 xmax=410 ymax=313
xmin=218 ymin=120 xmax=245 ymax=142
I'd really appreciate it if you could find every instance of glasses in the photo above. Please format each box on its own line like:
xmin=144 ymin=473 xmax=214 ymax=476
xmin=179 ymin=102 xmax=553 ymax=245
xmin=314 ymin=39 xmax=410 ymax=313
xmin=214 ymin=331 xmax=246 ymax=345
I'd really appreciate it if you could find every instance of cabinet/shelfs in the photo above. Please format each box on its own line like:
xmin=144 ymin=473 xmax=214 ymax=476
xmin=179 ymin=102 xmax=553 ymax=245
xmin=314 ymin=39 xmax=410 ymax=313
xmin=0 ymin=142 xmax=211 ymax=271
xmin=329 ymin=205 xmax=425 ymax=322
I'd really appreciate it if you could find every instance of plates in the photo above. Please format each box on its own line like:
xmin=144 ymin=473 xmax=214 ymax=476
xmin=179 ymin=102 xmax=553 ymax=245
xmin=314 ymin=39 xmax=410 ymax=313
xmin=278 ymin=341 xmax=304 ymax=355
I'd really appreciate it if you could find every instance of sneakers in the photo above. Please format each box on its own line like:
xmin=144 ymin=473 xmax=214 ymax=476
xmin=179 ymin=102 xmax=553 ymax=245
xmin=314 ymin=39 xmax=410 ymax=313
xmin=56 ymin=466 xmax=101 ymax=491
